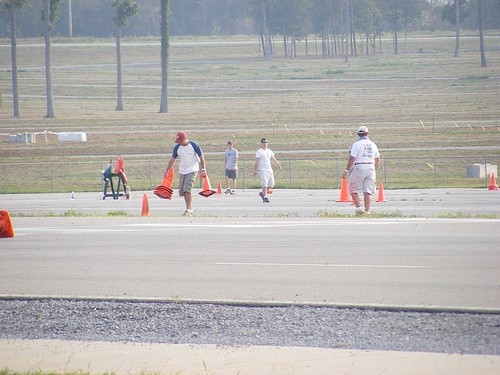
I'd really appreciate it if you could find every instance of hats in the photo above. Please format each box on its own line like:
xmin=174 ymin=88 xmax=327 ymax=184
xmin=228 ymin=141 xmax=233 ymax=144
xmin=357 ymin=126 xmax=368 ymax=134
xmin=175 ymin=132 xmax=185 ymax=143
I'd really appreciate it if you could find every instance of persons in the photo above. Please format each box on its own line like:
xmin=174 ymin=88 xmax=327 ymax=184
xmin=224 ymin=140 xmax=238 ymax=194
xmin=343 ymin=126 xmax=380 ymax=215
xmin=252 ymin=138 xmax=282 ymax=203
xmin=163 ymin=132 xmax=206 ymax=216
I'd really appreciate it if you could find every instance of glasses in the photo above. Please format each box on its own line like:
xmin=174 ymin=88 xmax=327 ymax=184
xmin=262 ymin=142 xmax=268 ymax=143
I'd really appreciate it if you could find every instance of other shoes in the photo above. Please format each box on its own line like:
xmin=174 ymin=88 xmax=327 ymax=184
xmin=355 ymin=207 xmax=369 ymax=215
xmin=182 ymin=209 xmax=195 ymax=217
xmin=225 ymin=189 xmax=231 ymax=194
xmin=262 ymin=196 xmax=269 ymax=203
xmin=259 ymin=192 xmax=264 ymax=198
xmin=230 ymin=190 xmax=236 ymax=194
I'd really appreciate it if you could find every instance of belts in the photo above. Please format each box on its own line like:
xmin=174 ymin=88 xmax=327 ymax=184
xmin=355 ymin=163 xmax=370 ymax=165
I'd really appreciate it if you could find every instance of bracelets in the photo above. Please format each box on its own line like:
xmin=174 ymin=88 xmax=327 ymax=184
xmin=202 ymin=169 xmax=205 ymax=170
xmin=234 ymin=164 xmax=236 ymax=165
xmin=345 ymin=170 xmax=348 ymax=172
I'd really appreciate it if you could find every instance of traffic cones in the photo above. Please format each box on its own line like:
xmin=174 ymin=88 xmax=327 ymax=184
xmin=488 ymin=171 xmax=499 ymax=190
xmin=375 ymin=183 xmax=386 ymax=203
xmin=336 ymin=175 xmax=353 ymax=202
xmin=141 ymin=193 xmax=149 ymax=217
xmin=198 ymin=174 xmax=216 ymax=197
xmin=217 ymin=180 xmax=221 ymax=194
xmin=114 ymin=154 xmax=126 ymax=175
xmin=154 ymin=167 xmax=174 ymax=200
